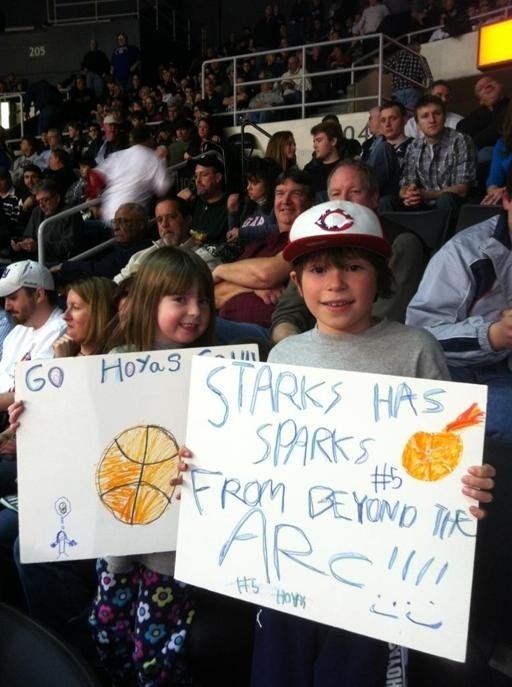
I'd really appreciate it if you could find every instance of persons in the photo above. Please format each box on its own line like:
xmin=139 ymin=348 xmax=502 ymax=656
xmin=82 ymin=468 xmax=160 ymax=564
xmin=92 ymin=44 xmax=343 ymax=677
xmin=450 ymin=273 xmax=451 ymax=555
xmin=168 ymin=195 xmax=499 ymax=687
xmin=0 ymin=1 xmax=512 ymax=641
xmin=5 ymin=247 xmax=230 ymax=687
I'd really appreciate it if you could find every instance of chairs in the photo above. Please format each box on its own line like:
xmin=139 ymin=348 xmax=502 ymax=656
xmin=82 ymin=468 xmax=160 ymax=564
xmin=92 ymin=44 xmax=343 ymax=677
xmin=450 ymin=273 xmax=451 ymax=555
xmin=455 ymin=200 xmax=505 ymax=239
xmin=378 ymin=202 xmax=449 ymax=275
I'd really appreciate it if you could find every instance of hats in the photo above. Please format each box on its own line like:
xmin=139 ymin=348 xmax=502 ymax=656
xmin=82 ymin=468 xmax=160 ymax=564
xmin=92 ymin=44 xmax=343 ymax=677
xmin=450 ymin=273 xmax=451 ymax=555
xmin=191 ymin=155 xmax=224 ymax=173
xmin=283 ymin=200 xmax=392 ymax=261
xmin=0 ymin=259 xmax=55 ymax=297
xmin=104 ymin=116 xmax=117 ymax=124
